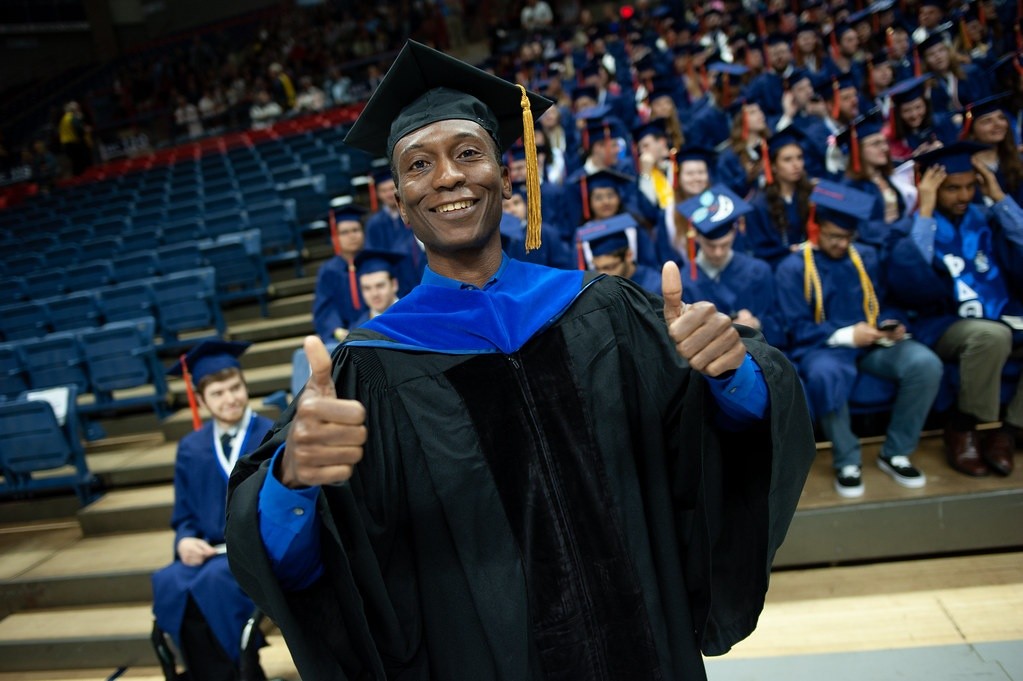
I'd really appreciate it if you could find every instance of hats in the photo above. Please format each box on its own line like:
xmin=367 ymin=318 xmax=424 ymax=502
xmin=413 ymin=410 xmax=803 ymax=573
xmin=168 ymin=341 xmax=256 ymax=433
xmin=675 ymin=184 xmax=753 ymax=281
xmin=494 ymin=0 xmax=1023 ymax=217
xmin=905 ymin=138 xmax=994 ymax=210
xmin=363 ymin=170 xmax=398 ymax=211
xmin=343 ymin=250 xmax=405 ymax=309
xmin=805 ymin=180 xmax=878 ymax=244
xmin=570 ymin=212 xmax=637 ymax=271
xmin=318 ymin=203 xmax=370 ymax=253
xmin=342 ymin=40 xmax=555 ymax=249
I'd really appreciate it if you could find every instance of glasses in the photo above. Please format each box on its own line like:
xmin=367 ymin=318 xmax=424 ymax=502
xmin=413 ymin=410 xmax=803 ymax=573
xmin=863 ymin=139 xmax=888 ymax=147
xmin=594 ymin=260 xmax=622 ymax=271
xmin=820 ymin=231 xmax=859 ymax=241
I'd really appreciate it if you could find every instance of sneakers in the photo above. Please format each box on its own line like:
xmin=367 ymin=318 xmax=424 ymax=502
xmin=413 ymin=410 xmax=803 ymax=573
xmin=876 ymin=455 xmax=926 ymax=488
xmin=833 ymin=464 xmax=865 ymax=498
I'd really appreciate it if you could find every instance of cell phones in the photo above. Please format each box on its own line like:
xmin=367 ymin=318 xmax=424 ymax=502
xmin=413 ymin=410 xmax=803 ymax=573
xmin=877 ymin=321 xmax=899 ymax=331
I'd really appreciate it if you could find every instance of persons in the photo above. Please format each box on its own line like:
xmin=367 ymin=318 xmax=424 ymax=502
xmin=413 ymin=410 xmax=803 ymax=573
xmin=222 ymin=35 xmax=818 ymax=681
xmin=884 ymin=139 xmax=1023 ymax=477
xmin=0 ymin=0 xmax=415 ymax=198
xmin=310 ymin=0 xmax=1023 ymax=362
xmin=149 ymin=336 xmax=278 ymax=681
xmin=774 ymin=178 xmax=944 ymax=501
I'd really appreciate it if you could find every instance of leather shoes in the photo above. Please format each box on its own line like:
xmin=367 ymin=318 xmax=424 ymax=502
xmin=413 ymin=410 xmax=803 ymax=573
xmin=948 ymin=426 xmax=1014 ymax=476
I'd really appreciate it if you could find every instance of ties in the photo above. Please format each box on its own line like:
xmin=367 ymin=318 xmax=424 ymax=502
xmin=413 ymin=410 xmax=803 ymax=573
xmin=221 ymin=434 xmax=234 ymax=460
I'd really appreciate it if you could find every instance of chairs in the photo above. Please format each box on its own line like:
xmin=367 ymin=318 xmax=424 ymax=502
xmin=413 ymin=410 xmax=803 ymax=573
xmin=0 ymin=100 xmax=384 ymax=507
